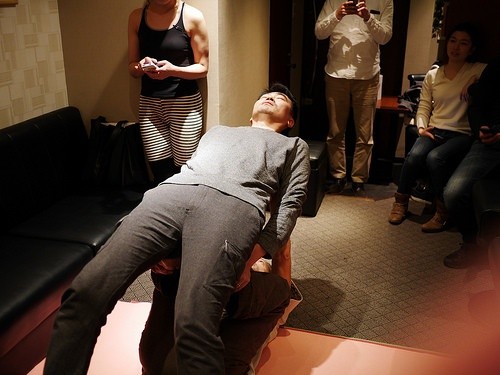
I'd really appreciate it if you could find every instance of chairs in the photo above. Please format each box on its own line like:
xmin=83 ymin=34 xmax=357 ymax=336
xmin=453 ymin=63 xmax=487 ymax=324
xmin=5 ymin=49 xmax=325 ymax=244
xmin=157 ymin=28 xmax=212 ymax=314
xmin=405 ymin=74 xmax=427 ymax=162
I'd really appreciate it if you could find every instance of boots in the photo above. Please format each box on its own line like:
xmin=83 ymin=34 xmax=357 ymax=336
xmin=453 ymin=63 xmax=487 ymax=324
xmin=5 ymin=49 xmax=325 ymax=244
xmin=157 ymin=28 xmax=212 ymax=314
xmin=422 ymin=210 xmax=448 ymax=234
xmin=389 ymin=193 xmax=410 ymax=225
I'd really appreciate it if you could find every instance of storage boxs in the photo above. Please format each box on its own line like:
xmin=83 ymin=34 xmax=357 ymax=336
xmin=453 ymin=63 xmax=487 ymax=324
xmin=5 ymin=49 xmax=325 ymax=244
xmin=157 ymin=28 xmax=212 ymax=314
xmin=298 ymin=142 xmax=326 ymax=216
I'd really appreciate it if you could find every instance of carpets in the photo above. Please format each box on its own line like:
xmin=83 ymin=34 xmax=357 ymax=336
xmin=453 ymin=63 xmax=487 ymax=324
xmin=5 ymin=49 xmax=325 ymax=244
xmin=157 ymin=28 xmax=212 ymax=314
xmin=114 ymin=183 xmax=466 ymax=356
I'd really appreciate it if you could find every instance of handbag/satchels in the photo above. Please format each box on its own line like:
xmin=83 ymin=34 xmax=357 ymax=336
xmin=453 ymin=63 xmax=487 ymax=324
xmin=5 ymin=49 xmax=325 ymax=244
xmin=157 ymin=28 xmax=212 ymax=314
xmin=90 ymin=116 xmax=149 ymax=195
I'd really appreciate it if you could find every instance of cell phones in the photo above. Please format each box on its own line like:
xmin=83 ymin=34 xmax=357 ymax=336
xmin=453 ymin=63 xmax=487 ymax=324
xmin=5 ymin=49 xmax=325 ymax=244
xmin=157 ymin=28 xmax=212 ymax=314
xmin=480 ymin=128 xmax=495 ymax=138
xmin=142 ymin=63 xmax=159 ymax=69
xmin=348 ymin=0 xmax=358 ymax=10
xmin=435 ymin=134 xmax=445 ymax=140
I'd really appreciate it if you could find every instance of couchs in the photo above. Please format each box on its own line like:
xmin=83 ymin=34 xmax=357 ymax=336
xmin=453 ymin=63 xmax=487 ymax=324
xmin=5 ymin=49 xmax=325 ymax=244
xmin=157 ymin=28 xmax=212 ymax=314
xmin=0 ymin=105 xmax=144 ymax=375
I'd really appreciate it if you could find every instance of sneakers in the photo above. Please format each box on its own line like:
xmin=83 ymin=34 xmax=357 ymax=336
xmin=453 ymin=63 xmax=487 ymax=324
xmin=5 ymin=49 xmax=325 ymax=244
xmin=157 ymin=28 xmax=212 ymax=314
xmin=352 ymin=182 xmax=366 ymax=198
xmin=444 ymin=243 xmax=484 ymax=268
xmin=334 ymin=177 xmax=347 ymax=196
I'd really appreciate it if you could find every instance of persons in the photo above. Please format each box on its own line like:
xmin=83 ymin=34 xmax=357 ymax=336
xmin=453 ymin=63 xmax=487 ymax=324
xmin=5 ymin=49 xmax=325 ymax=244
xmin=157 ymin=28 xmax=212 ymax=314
xmin=128 ymin=0 xmax=209 ymax=183
xmin=314 ymin=0 xmax=394 ymax=197
xmin=388 ymin=28 xmax=488 ymax=232
xmin=42 ymin=83 xmax=311 ymax=375
xmin=443 ymin=50 xmax=500 ymax=268
xmin=139 ymin=190 xmax=304 ymax=375
